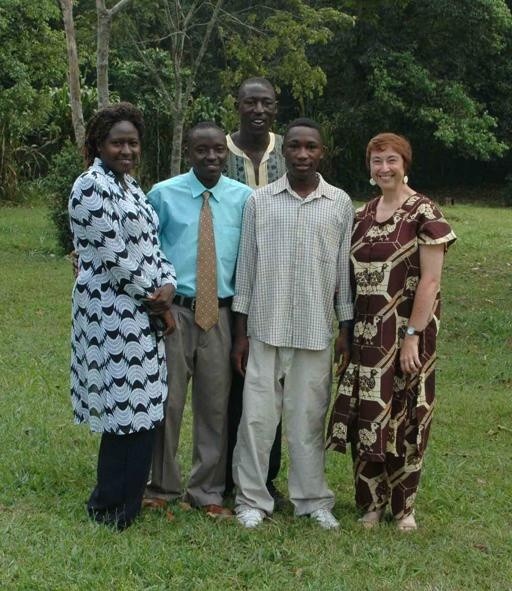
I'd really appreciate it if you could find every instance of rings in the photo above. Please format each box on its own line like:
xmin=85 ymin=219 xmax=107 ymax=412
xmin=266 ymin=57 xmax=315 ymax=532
xmin=410 ymin=361 xmax=414 ymax=366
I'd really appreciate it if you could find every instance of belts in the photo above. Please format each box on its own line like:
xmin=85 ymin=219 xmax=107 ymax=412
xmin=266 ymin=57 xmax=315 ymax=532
xmin=173 ymin=295 xmax=233 ymax=311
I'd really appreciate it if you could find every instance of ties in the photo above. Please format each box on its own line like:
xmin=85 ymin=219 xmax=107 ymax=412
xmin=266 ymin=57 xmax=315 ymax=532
xmin=195 ymin=192 xmax=219 ymax=332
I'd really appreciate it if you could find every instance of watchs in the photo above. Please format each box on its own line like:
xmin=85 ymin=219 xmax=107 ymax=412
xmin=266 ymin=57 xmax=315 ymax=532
xmin=405 ymin=325 xmax=423 ymax=337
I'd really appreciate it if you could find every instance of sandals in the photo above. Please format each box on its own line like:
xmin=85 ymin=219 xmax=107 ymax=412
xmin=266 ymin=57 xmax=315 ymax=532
xmin=361 ymin=511 xmax=380 ymax=530
xmin=398 ymin=515 xmax=417 ymax=533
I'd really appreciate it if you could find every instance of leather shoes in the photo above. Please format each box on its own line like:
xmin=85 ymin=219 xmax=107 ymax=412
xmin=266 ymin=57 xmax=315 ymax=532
xmin=143 ymin=497 xmax=167 ymax=510
xmin=206 ymin=505 xmax=233 ymax=518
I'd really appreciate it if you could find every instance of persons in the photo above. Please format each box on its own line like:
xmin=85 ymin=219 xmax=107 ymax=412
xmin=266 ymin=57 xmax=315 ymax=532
xmin=231 ymin=118 xmax=355 ymax=530
xmin=325 ymin=132 xmax=457 ymax=533
xmin=146 ymin=122 xmax=253 ymax=518
xmin=222 ymin=77 xmax=287 ymax=510
xmin=67 ymin=101 xmax=178 ymax=529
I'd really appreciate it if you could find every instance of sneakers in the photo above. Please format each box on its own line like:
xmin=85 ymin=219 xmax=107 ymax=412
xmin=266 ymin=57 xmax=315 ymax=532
xmin=310 ymin=509 xmax=339 ymax=530
xmin=236 ymin=509 xmax=265 ymax=529
xmin=267 ymin=483 xmax=284 ymax=499
